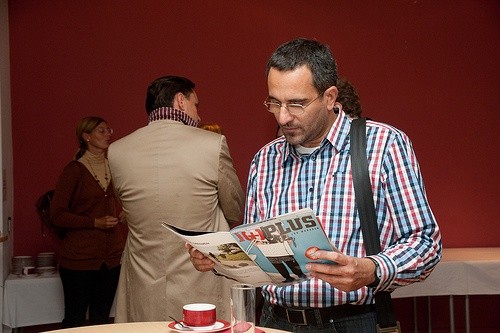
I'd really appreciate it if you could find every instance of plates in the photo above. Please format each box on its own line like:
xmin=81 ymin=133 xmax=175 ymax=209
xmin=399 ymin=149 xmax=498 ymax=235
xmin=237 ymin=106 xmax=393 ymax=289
xmin=168 ymin=317 xmax=235 ymax=333
xmin=12 ymin=252 xmax=56 ymax=275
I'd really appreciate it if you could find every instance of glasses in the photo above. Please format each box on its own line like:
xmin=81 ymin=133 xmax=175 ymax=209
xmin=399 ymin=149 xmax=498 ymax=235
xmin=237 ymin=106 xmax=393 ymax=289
xmin=96 ymin=128 xmax=113 ymax=134
xmin=263 ymin=92 xmax=324 ymax=115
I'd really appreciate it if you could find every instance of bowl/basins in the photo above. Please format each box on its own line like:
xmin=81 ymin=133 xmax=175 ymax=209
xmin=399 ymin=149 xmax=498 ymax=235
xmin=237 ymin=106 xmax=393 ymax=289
xmin=37 ymin=266 xmax=56 ymax=274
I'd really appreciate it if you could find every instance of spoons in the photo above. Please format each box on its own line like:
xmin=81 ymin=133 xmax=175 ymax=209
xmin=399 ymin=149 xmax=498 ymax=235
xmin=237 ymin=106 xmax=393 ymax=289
xmin=170 ymin=317 xmax=193 ymax=330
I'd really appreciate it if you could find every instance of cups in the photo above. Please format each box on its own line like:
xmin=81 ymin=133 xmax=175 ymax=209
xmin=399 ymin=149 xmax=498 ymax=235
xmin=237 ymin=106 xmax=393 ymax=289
xmin=22 ymin=267 xmax=35 ymax=276
xmin=182 ymin=303 xmax=217 ymax=327
xmin=228 ymin=284 xmax=256 ymax=333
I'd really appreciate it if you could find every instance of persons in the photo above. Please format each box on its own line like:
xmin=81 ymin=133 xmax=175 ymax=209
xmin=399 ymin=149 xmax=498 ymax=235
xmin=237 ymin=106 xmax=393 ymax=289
xmin=48 ymin=116 xmax=127 ymax=329
xmin=185 ymin=38 xmax=444 ymax=333
xmin=106 ymin=75 xmax=245 ymax=323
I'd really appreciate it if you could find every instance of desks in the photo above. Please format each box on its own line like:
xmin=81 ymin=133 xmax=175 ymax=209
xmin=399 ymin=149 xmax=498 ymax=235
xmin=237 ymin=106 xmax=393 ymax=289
xmin=1 ymin=270 xmax=116 ymax=333
xmin=392 ymin=247 xmax=500 ymax=333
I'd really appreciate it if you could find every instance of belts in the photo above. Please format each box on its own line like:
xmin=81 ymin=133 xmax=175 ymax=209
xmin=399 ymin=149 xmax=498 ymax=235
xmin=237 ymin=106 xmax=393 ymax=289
xmin=273 ymin=303 xmax=375 ymax=326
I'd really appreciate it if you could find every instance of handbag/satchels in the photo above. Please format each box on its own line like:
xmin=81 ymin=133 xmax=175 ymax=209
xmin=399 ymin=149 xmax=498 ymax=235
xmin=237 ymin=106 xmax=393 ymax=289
xmin=34 ymin=160 xmax=83 ymax=240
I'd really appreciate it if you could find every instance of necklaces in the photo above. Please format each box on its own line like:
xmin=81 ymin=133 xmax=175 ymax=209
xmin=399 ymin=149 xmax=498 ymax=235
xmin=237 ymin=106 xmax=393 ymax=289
xmin=84 ymin=156 xmax=109 ymax=190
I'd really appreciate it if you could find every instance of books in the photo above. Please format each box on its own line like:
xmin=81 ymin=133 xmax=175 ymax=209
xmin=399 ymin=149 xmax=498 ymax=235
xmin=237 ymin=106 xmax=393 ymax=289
xmin=159 ymin=208 xmax=342 ymax=289
